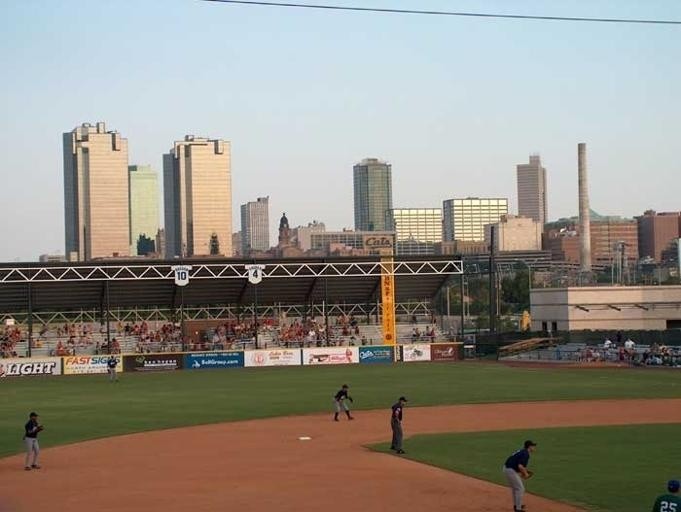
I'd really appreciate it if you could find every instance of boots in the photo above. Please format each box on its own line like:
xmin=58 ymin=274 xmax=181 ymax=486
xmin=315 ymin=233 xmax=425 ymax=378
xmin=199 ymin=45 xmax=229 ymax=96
xmin=334 ymin=410 xmax=354 ymax=422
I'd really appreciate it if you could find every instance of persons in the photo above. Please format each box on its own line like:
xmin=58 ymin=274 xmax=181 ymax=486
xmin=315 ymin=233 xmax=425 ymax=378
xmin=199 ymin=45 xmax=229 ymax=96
xmin=108 ymin=356 xmax=118 ymax=381
xmin=334 ymin=384 xmax=354 ymax=421
xmin=413 ymin=325 xmax=436 ymax=343
xmin=503 ymin=440 xmax=537 ymax=512
xmin=1 ymin=313 xmax=368 ymax=358
xmin=653 ymin=480 xmax=681 ymax=512
xmin=24 ymin=412 xmax=43 ymax=470
xmin=390 ymin=397 xmax=408 ymax=454
xmin=575 ymin=337 xmax=681 ymax=368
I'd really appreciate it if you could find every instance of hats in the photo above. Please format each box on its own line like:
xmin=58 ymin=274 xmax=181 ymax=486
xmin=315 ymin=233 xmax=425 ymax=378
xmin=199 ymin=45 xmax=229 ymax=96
xmin=342 ymin=384 xmax=350 ymax=389
xmin=30 ymin=412 xmax=39 ymax=417
xmin=525 ymin=441 xmax=538 ymax=448
xmin=399 ymin=397 xmax=409 ymax=402
xmin=667 ymin=479 xmax=681 ymax=489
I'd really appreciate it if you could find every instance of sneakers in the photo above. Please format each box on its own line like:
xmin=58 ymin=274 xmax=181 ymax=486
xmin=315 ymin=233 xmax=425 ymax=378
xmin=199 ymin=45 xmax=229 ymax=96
xmin=513 ymin=504 xmax=527 ymax=512
xmin=25 ymin=465 xmax=40 ymax=470
xmin=391 ymin=445 xmax=405 ymax=453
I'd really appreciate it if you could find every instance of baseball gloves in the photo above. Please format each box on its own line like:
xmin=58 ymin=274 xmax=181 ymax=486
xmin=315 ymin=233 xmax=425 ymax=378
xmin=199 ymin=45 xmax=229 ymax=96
xmin=107 ymin=369 xmax=111 ymax=374
xmin=520 ymin=470 xmax=534 ymax=480
xmin=37 ymin=425 xmax=46 ymax=431
xmin=348 ymin=397 xmax=353 ymax=403
